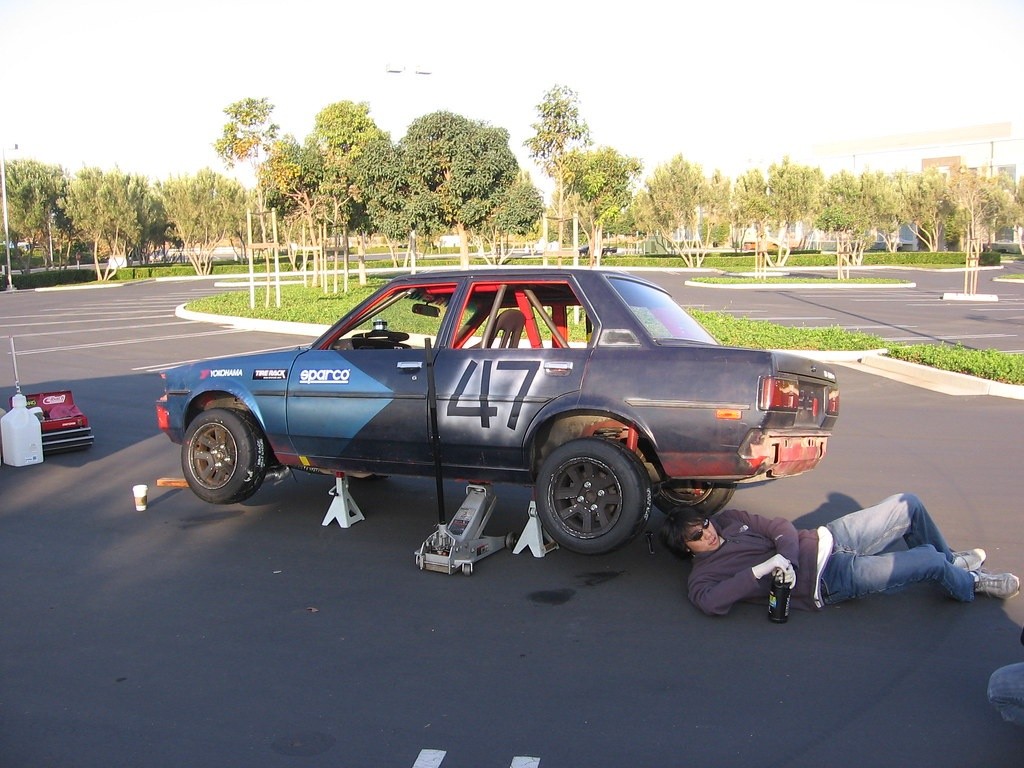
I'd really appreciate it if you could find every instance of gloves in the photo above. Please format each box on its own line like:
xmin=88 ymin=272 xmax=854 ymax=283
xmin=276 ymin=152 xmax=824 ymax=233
xmin=752 ymin=553 xmax=790 ymax=580
xmin=774 ymin=558 xmax=796 ymax=589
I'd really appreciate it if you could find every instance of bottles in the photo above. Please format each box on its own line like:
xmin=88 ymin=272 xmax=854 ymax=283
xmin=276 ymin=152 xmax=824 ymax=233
xmin=1 ymin=394 xmax=43 ymax=466
xmin=767 ymin=568 xmax=791 ymax=623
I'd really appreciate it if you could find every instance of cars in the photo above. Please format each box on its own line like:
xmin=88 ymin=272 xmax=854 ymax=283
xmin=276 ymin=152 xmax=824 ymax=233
xmin=154 ymin=267 xmax=844 ymax=559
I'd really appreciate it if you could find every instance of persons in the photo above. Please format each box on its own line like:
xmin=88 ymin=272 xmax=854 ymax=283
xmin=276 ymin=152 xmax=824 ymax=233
xmin=986 ymin=625 xmax=1024 ymax=727
xmin=659 ymin=492 xmax=1020 ymax=616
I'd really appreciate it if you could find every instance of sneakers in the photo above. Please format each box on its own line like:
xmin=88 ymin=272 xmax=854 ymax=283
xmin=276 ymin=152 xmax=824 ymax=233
xmin=970 ymin=571 xmax=1020 ymax=599
xmin=950 ymin=548 xmax=987 ymax=571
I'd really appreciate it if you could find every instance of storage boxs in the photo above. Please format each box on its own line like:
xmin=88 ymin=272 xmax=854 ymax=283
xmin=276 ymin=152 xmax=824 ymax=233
xmin=9 ymin=390 xmax=87 ymax=432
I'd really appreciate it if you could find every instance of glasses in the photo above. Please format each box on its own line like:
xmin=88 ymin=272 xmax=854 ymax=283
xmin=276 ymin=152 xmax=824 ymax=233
xmin=683 ymin=517 xmax=709 ymax=543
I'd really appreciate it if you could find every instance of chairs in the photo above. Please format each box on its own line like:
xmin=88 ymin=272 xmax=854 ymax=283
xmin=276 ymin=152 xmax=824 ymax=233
xmin=487 ymin=309 xmax=526 ymax=348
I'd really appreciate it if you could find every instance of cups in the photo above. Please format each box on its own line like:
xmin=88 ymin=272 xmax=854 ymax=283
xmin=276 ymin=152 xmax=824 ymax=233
xmin=132 ymin=484 xmax=149 ymax=512
